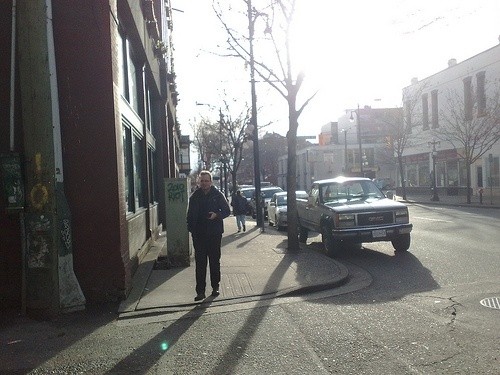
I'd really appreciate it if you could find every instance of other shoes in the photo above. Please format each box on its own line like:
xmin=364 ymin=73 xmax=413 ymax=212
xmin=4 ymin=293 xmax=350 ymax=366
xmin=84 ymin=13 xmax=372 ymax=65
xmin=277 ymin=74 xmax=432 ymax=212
xmin=195 ymin=293 xmax=205 ymax=301
xmin=212 ymin=285 xmax=219 ymax=296
xmin=243 ymin=225 xmax=245 ymax=231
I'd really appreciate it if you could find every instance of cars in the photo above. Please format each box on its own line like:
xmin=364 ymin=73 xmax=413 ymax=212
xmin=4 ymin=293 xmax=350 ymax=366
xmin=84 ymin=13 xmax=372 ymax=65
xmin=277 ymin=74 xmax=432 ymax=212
xmin=266 ymin=190 xmax=310 ymax=232
xmin=237 ymin=183 xmax=284 ymax=221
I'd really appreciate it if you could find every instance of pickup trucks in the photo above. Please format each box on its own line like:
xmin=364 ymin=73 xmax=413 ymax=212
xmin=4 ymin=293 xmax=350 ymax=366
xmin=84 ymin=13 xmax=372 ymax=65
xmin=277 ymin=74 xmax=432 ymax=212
xmin=296 ymin=176 xmax=413 ymax=259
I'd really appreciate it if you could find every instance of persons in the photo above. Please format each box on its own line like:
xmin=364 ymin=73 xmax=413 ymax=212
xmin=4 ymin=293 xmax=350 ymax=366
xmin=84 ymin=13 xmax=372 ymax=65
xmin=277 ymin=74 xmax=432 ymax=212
xmin=187 ymin=171 xmax=231 ymax=301
xmin=231 ymin=190 xmax=246 ymax=232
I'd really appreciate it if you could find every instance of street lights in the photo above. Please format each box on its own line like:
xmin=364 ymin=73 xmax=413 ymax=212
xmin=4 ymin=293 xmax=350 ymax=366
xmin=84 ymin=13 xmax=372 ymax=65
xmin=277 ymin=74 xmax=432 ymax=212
xmin=245 ymin=0 xmax=271 ymax=229
xmin=430 ymin=135 xmax=440 ymax=201
xmin=349 ymin=103 xmax=364 ymax=177
xmin=339 ymin=127 xmax=351 ymax=177
xmin=218 ymin=106 xmax=230 ymax=192
